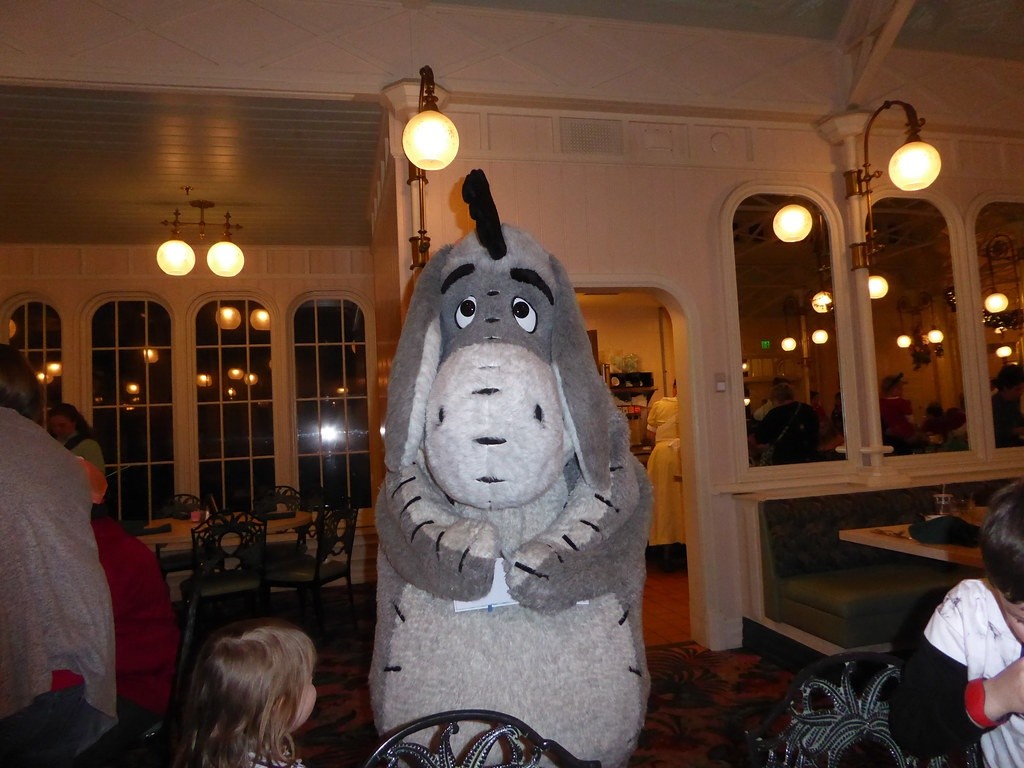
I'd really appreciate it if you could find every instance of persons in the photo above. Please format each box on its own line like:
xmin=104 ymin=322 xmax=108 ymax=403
xmin=0 ymin=341 xmax=183 ymax=768
xmin=887 ymin=479 xmax=1024 ymax=768
xmin=742 ymin=364 xmax=1024 ymax=468
xmin=162 ymin=618 xmax=317 ymax=768
xmin=646 ymin=378 xmax=688 ymax=570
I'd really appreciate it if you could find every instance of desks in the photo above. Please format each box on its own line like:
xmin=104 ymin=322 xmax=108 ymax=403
xmin=836 ymin=502 xmax=1000 ymax=581
xmin=129 ymin=512 xmax=324 ymax=562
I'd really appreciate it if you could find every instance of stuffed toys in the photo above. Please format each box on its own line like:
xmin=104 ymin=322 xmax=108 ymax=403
xmin=362 ymin=168 xmax=653 ymax=768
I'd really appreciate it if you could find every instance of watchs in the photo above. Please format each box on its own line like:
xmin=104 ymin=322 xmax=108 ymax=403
xmin=965 ymin=677 xmax=1009 ymax=727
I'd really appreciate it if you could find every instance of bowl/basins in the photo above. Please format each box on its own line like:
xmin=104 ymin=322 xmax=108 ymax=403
xmin=925 ymin=515 xmax=942 ymax=521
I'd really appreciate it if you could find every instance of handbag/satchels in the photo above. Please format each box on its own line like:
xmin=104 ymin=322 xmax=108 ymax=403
xmin=760 ymin=445 xmax=775 ymax=466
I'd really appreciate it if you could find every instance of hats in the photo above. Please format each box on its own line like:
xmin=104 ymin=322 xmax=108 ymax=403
xmin=942 ymin=407 xmax=966 ymax=434
xmin=75 ymin=456 xmax=108 ymax=505
xmin=909 ymin=515 xmax=982 ymax=548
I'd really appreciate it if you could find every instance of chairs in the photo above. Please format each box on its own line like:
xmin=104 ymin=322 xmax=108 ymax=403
xmin=158 ymin=485 xmax=360 ymax=627
xmin=122 ymin=586 xmax=202 ymax=748
xmin=735 ymin=641 xmax=981 ymax=768
xmin=359 ymin=708 xmax=603 ymax=768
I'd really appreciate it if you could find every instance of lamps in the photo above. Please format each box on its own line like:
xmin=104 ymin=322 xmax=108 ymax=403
xmin=398 ymin=63 xmax=462 ymax=270
xmin=897 ymin=291 xmax=945 ymax=371
xmin=868 ymin=272 xmax=892 ymax=306
xmin=155 ymin=199 xmax=246 ymax=280
xmin=846 ymin=94 xmax=945 ymax=270
xmin=766 ymin=204 xmax=836 ymax=359
xmin=984 ymin=285 xmax=1009 ymax=319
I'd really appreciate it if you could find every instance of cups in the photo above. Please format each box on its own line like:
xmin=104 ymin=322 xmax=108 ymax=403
xmin=952 ymin=500 xmax=976 ymax=525
xmin=191 ymin=512 xmax=201 ymax=521
xmin=601 ymin=349 xmax=623 ymax=374
xmin=623 ymin=352 xmax=639 ymax=373
xmin=933 ymin=494 xmax=954 ymax=515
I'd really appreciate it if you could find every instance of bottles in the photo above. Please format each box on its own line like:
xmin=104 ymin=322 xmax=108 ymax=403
xmin=603 ymin=364 xmax=610 ymax=385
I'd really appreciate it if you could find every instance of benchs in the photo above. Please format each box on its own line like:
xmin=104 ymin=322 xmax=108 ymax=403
xmin=740 ymin=471 xmax=1024 ymax=650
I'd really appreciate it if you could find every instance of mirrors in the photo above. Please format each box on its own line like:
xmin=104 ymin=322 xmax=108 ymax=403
xmin=964 ymin=193 xmax=1024 ymax=460
xmin=707 ymin=173 xmax=856 ymax=479
xmin=859 ymin=189 xmax=973 ymax=467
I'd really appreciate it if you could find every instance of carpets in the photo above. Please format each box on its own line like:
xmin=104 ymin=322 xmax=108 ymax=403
xmin=620 ymin=634 xmax=819 ymax=768
